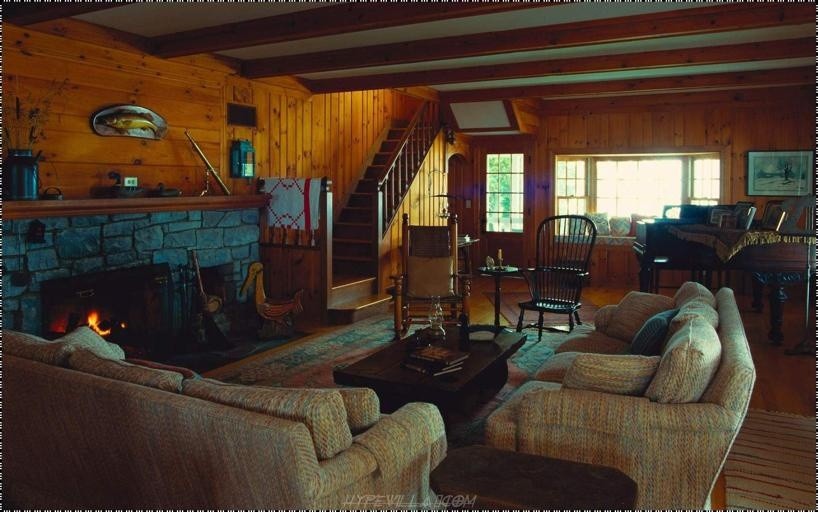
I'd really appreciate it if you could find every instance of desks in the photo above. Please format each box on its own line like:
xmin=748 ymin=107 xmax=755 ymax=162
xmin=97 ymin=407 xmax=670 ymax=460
xmin=477 ymin=265 xmax=519 ymax=330
xmin=430 ymin=446 xmax=639 ymax=512
xmin=457 ymin=235 xmax=481 ymax=292
xmin=664 ymin=219 xmax=817 ymax=347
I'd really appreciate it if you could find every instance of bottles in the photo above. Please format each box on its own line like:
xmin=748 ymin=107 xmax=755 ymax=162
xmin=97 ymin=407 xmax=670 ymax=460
xmin=429 ymin=295 xmax=443 ymax=336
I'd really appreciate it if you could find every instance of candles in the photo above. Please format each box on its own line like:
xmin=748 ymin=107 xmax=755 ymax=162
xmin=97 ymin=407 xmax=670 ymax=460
xmin=498 ymin=249 xmax=503 ymax=259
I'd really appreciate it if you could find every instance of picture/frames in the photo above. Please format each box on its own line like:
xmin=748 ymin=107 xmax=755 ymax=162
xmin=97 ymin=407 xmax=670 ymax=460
xmin=704 ymin=200 xmax=817 ymax=234
xmin=747 ymin=150 xmax=815 ymax=196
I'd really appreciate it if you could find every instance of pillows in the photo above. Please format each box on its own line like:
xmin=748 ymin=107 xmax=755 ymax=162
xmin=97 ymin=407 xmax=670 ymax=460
xmin=568 ymin=209 xmax=656 ymax=238
xmin=562 ymin=278 xmax=724 ymax=405
xmin=404 ymin=252 xmax=457 ymax=300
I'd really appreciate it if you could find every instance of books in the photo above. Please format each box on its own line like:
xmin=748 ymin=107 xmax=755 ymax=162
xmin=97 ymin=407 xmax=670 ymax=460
xmin=457 ymin=232 xmax=471 ymax=244
xmin=399 ymin=358 xmax=464 ymax=377
xmin=409 ymin=342 xmax=472 ymax=366
xmin=413 ymin=357 xmax=465 ymax=371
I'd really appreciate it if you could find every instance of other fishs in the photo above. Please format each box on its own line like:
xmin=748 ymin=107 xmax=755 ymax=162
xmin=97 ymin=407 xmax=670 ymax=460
xmin=97 ymin=108 xmax=163 ymax=138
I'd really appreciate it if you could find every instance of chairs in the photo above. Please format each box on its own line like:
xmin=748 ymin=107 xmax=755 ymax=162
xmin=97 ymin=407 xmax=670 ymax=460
xmin=388 ymin=211 xmax=473 ymax=340
xmin=516 ymin=214 xmax=597 ymax=342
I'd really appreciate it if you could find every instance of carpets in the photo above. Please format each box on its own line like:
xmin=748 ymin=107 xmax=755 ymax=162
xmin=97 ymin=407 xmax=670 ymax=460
xmin=724 ymin=407 xmax=816 ymax=510
xmin=201 ymin=313 xmax=596 ymax=445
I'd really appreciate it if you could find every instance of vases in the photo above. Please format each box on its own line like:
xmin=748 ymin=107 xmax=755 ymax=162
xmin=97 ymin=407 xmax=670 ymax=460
xmin=1 ymin=147 xmax=39 ymax=202
xmin=428 ymin=293 xmax=447 ymax=338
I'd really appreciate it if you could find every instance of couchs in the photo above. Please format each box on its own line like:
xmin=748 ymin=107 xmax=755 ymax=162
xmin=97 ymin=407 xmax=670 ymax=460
xmin=485 ymin=286 xmax=756 ymax=511
xmin=0 ymin=327 xmax=448 ymax=511
